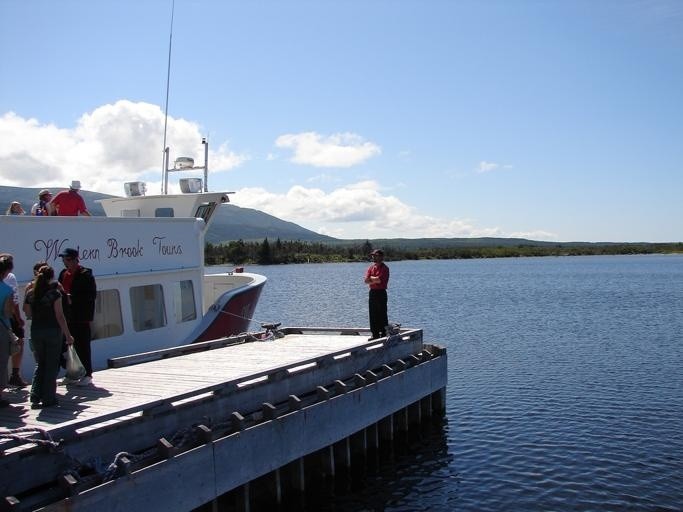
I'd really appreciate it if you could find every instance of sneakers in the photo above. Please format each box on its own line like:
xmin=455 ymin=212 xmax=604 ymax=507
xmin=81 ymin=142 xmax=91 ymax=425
xmin=366 ymin=333 xmax=380 ymax=341
xmin=56 ymin=375 xmax=78 ymax=386
xmin=9 ymin=375 xmax=28 ymax=387
xmin=75 ymin=375 xmax=93 ymax=387
xmin=30 ymin=395 xmax=60 ymax=409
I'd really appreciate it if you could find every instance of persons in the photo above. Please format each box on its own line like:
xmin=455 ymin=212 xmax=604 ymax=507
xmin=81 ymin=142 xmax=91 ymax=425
xmin=31 ymin=189 xmax=53 ymax=216
xmin=49 ymin=181 xmax=92 ymax=216
xmin=364 ymin=248 xmax=389 ymax=342
xmin=0 ymin=256 xmax=16 ymax=407
xmin=24 ymin=261 xmax=49 ymax=364
xmin=56 ymin=248 xmax=97 ymax=387
xmin=23 ymin=266 xmax=75 ymax=406
xmin=1 ymin=253 xmax=28 ymax=387
xmin=7 ymin=201 xmax=27 ymax=216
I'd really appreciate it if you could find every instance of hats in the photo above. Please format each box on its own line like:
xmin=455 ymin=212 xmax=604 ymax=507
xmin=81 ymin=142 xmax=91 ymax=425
xmin=370 ymin=248 xmax=384 ymax=257
xmin=68 ymin=180 xmax=83 ymax=190
xmin=38 ymin=189 xmax=53 ymax=197
xmin=57 ymin=247 xmax=80 ymax=258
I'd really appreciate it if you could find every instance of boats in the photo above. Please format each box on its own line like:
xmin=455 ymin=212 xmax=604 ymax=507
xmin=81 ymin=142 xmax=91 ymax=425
xmin=1 ymin=132 xmax=269 ymax=386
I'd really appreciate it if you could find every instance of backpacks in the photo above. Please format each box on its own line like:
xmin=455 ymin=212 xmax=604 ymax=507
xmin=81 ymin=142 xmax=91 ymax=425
xmin=7 ymin=329 xmax=22 ymax=358
